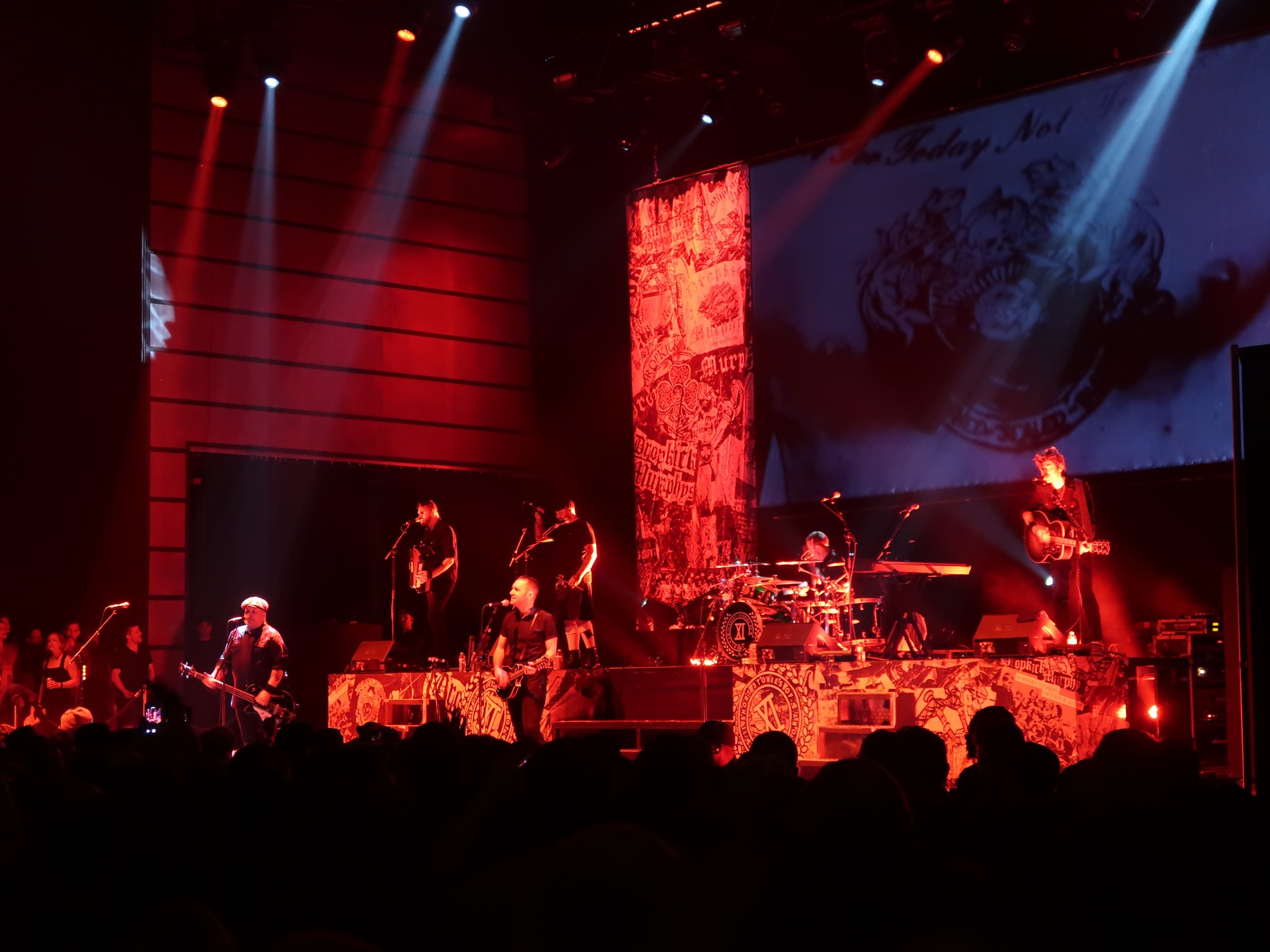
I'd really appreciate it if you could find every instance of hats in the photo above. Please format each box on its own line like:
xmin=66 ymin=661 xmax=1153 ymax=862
xmin=241 ymin=596 xmax=269 ymax=611
xmin=61 ymin=706 xmax=93 ymax=732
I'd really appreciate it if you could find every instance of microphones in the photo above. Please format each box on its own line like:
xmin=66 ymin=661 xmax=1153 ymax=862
xmin=405 ymin=516 xmax=422 ymax=525
xmin=105 ymin=601 xmax=129 ymax=609
xmin=899 ymin=504 xmax=919 ymax=512
xmin=553 ymin=517 xmax=570 ymax=527
xmin=821 ymin=491 xmax=841 ymax=502
xmin=487 ymin=599 xmax=509 ymax=607
xmin=1033 ymin=475 xmax=1048 ymax=481
xmin=229 ymin=615 xmax=249 ymax=622
xmin=427 ymin=657 xmax=445 ymax=663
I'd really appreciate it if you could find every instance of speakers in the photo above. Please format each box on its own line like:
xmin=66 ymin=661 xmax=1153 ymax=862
xmin=972 ymin=612 xmax=1048 ymax=658
xmin=756 ymin=622 xmax=816 ymax=663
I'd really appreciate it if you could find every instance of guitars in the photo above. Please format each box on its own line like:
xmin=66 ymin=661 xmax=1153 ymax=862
xmin=495 ymin=649 xmax=565 ymax=700
xmin=1023 ymin=508 xmax=1111 ymax=564
xmin=177 ymin=661 xmax=299 ymax=728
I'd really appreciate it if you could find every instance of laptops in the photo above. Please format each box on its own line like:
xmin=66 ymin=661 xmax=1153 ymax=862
xmin=352 ymin=640 xmax=398 ymax=662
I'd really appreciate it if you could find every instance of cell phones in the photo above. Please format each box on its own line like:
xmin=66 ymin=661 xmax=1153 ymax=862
xmin=144 ymin=701 xmax=163 ymax=733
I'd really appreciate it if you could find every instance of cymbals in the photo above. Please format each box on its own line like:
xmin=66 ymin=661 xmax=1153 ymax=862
xmin=774 ymin=559 xmax=824 ymax=566
xmin=743 ymin=574 xmax=774 ymax=581
xmin=715 ymin=561 xmax=771 ymax=569
xmin=745 ymin=577 xmax=803 ymax=588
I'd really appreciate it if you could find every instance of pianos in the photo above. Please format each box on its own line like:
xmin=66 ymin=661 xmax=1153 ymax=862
xmin=852 ymin=554 xmax=972 ymax=577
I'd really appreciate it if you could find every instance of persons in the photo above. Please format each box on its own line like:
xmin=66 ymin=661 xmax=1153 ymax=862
xmin=202 ymin=596 xmax=289 ymax=746
xmin=409 ymin=501 xmax=459 ymax=670
xmin=389 ymin=607 xmax=425 ymax=672
xmin=805 ymin=530 xmax=850 ymax=636
xmin=0 ymin=702 xmax=1270 ymax=952
xmin=1022 ymin=446 xmax=1105 ymax=656
xmin=110 ymin=622 xmax=156 ymax=729
xmin=186 ymin=615 xmax=221 ymax=728
xmin=493 ymin=576 xmax=558 ymax=757
xmin=535 ymin=498 xmax=602 ymax=669
xmin=1 ymin=615 xmax=110 ymax=702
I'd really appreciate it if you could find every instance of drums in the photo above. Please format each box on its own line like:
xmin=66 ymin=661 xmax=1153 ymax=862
xmin=713 ymin=598 xmax=795 ymax=662
